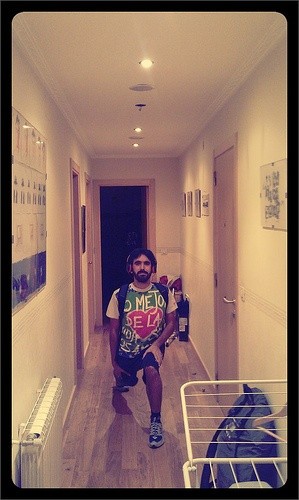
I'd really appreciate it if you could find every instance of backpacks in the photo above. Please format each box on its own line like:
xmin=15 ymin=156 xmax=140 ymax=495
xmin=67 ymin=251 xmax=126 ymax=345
xmin=198 ymin=383 xmax=277 ymax=489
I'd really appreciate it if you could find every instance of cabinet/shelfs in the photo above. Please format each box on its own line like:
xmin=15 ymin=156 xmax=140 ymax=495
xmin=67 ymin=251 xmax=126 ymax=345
xmin=179 ymin=378 xmax=287 ymax=488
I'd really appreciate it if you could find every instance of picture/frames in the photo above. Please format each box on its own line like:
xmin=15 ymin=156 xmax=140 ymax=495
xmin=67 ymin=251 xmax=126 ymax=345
xmin=257 ymin=158 xmax=287 ymax=232
xmin=179 ymin=188 xmax=212 ymax=221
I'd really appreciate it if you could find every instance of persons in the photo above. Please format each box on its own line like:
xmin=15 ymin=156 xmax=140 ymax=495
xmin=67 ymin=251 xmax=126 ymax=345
xmin=102 ymin=246 xmax=180 ymax=448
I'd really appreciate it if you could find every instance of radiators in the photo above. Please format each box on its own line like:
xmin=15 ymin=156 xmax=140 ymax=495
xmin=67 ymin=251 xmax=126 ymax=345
xmin=16 ymin=375 xmax=63 ymax=487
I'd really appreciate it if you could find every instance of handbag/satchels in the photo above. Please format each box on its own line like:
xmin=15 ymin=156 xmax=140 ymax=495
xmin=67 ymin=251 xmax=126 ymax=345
xmin=159 ymin=274 xmax=182 ymax=292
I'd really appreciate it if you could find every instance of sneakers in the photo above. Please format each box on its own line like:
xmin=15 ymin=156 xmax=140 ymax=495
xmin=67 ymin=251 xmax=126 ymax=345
xmin=114 ymin=381 xmax=131 ymax=391
xmin=148 ymin=418 xmax=165 ymax=449
